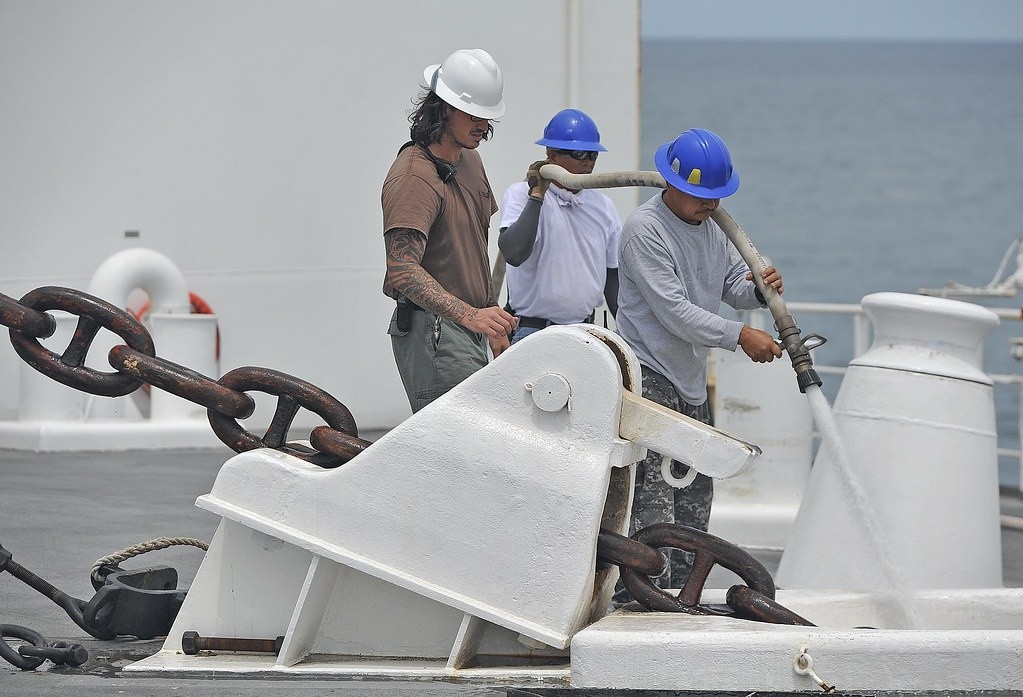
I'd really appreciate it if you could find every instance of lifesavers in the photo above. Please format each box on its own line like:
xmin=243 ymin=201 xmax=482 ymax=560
xmin=127 ymin=291 xmax=223 ymax=397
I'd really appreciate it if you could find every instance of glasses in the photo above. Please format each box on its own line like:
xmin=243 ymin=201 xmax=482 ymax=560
xmin=453 ymin=108 xmax=482 ymax=122
xmin=548 ymin=147 xmax=598 ymax=162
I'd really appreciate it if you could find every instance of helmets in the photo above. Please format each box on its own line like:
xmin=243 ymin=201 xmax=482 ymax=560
xmin=424 ymin=49 xmax=505 ymax=120
xmin=655 ymin=128 xmax=741 ymax=198
xmin=534 ymin=109 xmax=608 ymax=151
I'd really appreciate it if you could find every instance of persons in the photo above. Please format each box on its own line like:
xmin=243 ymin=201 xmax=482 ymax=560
xmin=380 ymin=48 xmax=519 ymax=415
xmin=611 ymin=127 xmax=784 ymax=610
xmin=499 ymin=109 xmax=623 ymax=348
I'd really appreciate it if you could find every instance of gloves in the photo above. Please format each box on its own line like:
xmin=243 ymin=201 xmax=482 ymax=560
xmin=528 ymin=161 xmax=552 ymax=195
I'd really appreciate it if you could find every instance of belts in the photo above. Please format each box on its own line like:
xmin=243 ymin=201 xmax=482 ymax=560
xmin=518 ymin=316 xmax=555 ymax=329
